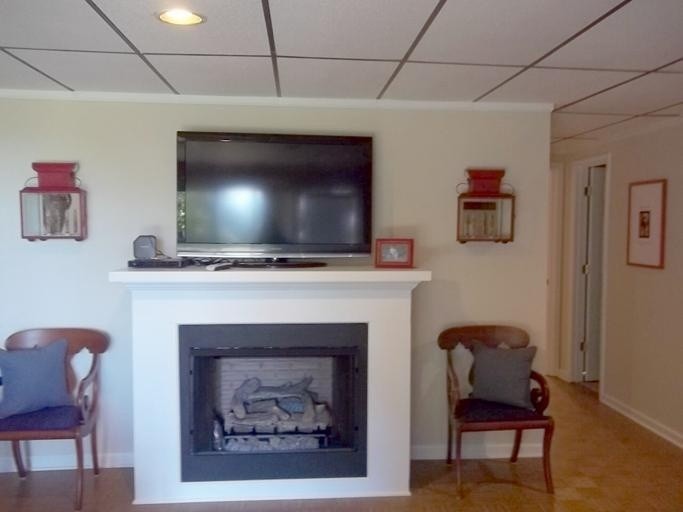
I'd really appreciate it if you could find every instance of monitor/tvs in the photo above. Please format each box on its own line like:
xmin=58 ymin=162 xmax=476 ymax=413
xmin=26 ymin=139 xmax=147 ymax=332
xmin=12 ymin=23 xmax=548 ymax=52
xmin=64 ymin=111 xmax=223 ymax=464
xmin=176 ymin=131 xmax=372 ymax=268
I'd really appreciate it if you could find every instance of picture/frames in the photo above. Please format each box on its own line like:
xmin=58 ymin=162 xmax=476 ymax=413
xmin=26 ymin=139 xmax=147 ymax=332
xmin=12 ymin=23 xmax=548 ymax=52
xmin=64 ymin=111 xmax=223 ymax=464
xmin=626 ymin=179 xmax=667 ymax=268
xmin=375 ymin=238 xmax=414 ymax=268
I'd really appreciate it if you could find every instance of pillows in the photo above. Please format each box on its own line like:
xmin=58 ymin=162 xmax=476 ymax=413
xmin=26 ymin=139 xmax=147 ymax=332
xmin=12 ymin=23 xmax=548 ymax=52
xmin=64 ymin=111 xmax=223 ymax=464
xmin=469 ymin=334 xmax=538 ymax=411
xmin=0 ymin=335 xmax=73 ymax=421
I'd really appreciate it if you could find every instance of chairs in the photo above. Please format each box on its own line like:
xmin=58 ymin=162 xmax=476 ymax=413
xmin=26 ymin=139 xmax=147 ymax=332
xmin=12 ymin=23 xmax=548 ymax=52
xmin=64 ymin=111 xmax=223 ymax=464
xmin=0 ymin=328 xmax=108 ymax=511
xmin=437 ymin=326 xmax=555 ymax=499
